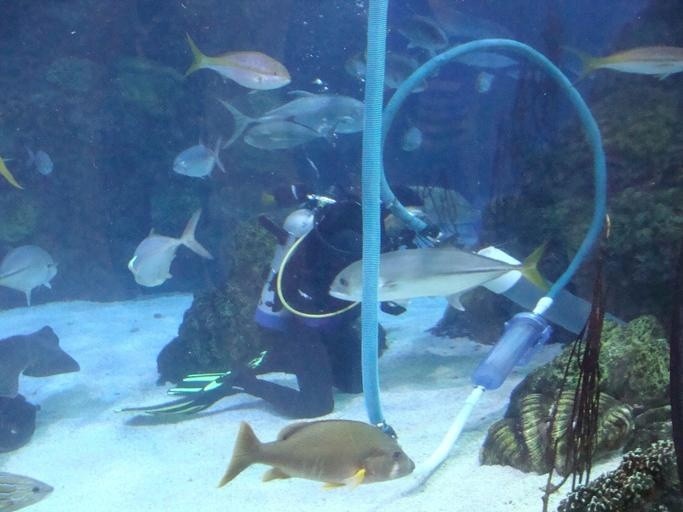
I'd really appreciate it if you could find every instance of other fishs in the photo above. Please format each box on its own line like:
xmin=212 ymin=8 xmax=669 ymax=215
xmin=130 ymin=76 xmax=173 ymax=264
xmin=344 ymin=7 xmax=544 ymax=180
xmin=241 ymin=122 xmax=342 ymax=149
xmin=215 ymin=93 xmax=364 ymax=148
xmin=173 ymin=138 xmax=228 ymax=179
xmin=182 ymin=35 xmax=293 ymax=90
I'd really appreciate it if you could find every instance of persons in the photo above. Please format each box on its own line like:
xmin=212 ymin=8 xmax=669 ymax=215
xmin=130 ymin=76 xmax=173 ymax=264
xmin=114 ymin=181 xmax=441 ymax=420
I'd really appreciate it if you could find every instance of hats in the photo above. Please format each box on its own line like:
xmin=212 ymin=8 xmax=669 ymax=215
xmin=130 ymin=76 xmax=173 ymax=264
xmin=380 ymin=184 xmax=422 ymax=212
